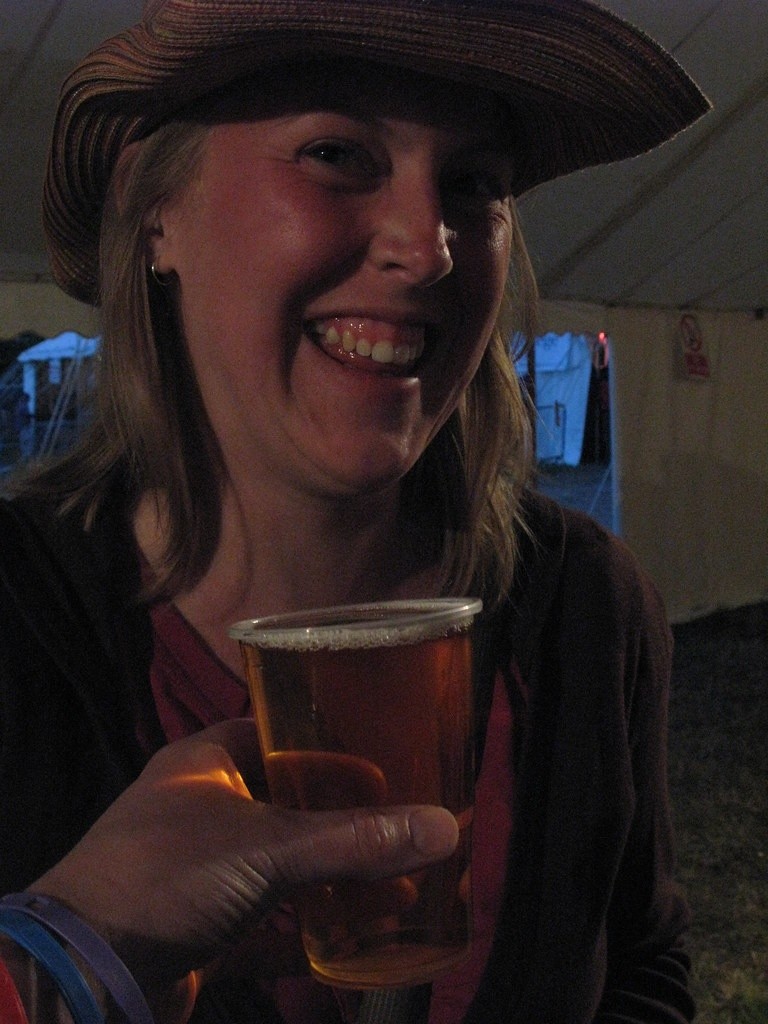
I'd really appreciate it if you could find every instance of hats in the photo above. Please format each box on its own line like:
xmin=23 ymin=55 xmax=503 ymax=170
xmin=39 ymin=0 xmax=711 ymax=309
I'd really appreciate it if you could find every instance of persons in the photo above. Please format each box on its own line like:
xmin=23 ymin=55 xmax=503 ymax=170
xmin=0 ymin=718 xmax=459 ymax=1024
xmin=0 ymin=0 xmax=715 ymax=1024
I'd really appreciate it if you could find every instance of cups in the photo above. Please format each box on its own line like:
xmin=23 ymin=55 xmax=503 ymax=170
xmin=230 ymin=597 xmax=484 ymax=990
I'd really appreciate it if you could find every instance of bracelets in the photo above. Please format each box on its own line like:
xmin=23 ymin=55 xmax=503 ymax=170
xmin=0 ymin=892 xmax=154 ymax=1024
